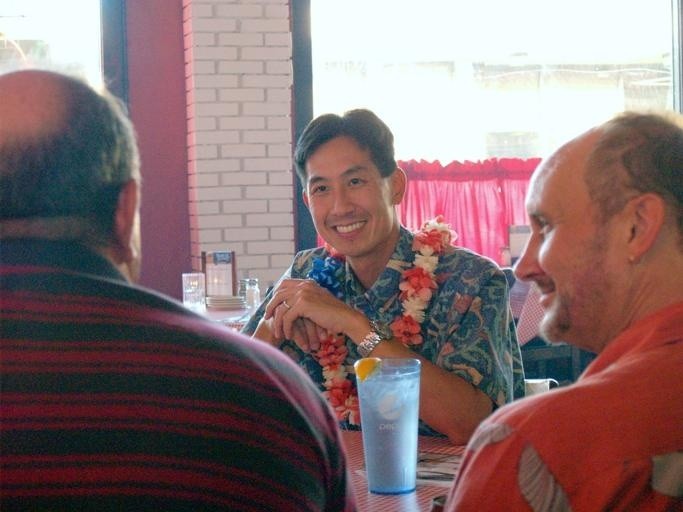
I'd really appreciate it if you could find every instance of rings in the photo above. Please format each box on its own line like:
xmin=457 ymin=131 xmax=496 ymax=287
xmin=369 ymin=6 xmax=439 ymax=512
xmin=283 ymin=300 xmax=290 ymax=309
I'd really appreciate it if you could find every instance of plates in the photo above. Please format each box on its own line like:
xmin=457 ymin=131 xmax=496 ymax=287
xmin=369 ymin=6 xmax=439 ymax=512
xmin=206 ymin=293 xmax=246 ymax=309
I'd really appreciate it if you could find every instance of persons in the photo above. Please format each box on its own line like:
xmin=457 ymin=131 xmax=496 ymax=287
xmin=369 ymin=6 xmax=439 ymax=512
xmin=1 ymin=67 xmax=362 ymax=512
xmin=239 ymin=107 xmax=526 ymax=447
xmin=444 ymin=106 xmax=683 ymax=512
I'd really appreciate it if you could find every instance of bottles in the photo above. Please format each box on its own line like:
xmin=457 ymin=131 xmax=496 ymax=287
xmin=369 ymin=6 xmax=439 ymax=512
xmin=206 ymin=253 xmax=235 ymax=297
xmin=500 ymin=246 xmax=512 ymax=265
xmin=239 ymin=276 xmax=260 ymax=305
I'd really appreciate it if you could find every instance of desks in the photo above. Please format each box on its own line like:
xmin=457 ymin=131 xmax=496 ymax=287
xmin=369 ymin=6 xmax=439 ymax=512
xmin=333 ymin=424 xmax=470 ymax=512
xmin=196 ymin=299 xmax=261 ymax=331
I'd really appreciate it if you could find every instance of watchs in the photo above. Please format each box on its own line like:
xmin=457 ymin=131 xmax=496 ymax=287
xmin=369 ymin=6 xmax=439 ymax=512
xmin=357 ymin=319 xmax=394 ymax=358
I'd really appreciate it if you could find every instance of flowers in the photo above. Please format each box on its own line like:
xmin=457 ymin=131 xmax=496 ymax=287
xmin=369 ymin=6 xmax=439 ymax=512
xmin=302 ymin=214 xmax=458 ymax=423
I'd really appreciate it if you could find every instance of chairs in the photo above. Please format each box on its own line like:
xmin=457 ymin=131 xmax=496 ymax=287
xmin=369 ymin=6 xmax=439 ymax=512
xmin=518 ymin=344 xmax=580 ymax=397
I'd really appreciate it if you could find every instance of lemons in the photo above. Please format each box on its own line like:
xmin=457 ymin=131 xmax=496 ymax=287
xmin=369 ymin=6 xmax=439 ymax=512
xmin=354 ymin=358 xmax=382 ymax=383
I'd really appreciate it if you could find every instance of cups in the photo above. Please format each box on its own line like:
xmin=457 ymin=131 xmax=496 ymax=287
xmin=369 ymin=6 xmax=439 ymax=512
xmin=181 ymin=273 xmax=205 ymax=311
xmin=524 ymin=379 xmax=559 ymax=400
xmin=352 ymin=358 xmax=422 ymax=495
